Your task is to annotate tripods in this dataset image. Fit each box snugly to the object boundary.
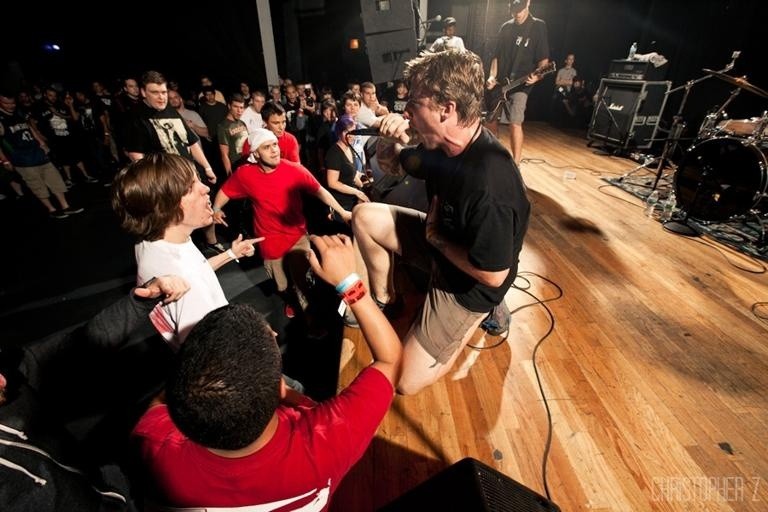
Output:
[616,64,733,192]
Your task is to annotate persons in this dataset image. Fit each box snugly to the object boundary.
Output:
[132,232,404,512]
[325,116,371,232]
[115,75,140,120]
[284,76,335,128]
[211,129,353,319]
[114,71,227,254]
[243,101,301,165]
[266,83,282,111]
[112,152,304,393]
[553,54,578,115]
[389,79,412,114]
[198,75,226,103]
[353,50,530,396]
[0,273,192,512]
[335,93,361,138]
[167,89,208,138]
[235,78,253,107]
[429,16,465,52]
[215,94,250,177]
[168,81,178,91]
[240,92,266,145]
[197,86,229,136]
[355,81,388,129]
[0,70,112,220]
[484,0,550,166]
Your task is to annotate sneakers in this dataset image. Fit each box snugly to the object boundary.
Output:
[484,300,511,332]
[66,178,75,185]
[62,205,84,215]
[284,303,295,319]
[83,175,99,183]
[49,211,69,219]
[207,242,226,255]
[342,291,407,328]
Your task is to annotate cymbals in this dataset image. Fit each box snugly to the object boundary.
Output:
[704,67,768,104]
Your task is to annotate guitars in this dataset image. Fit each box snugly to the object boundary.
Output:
[484,60,556,123]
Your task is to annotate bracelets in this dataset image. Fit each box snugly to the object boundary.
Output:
[212,208,221,211]
[204,167,213,171]
[337,273,359,293]
[338,281,368,304]
[227,248,237,260]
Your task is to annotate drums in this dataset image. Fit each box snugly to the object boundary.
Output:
[673,135,768,225]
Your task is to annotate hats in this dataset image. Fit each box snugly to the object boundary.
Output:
[506,1,530,13]
[440,16,456,26]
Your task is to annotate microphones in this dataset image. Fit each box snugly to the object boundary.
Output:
[421,14,442,24]
[348,127,420,146]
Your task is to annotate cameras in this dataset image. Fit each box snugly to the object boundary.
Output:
[304,89,315,107]
